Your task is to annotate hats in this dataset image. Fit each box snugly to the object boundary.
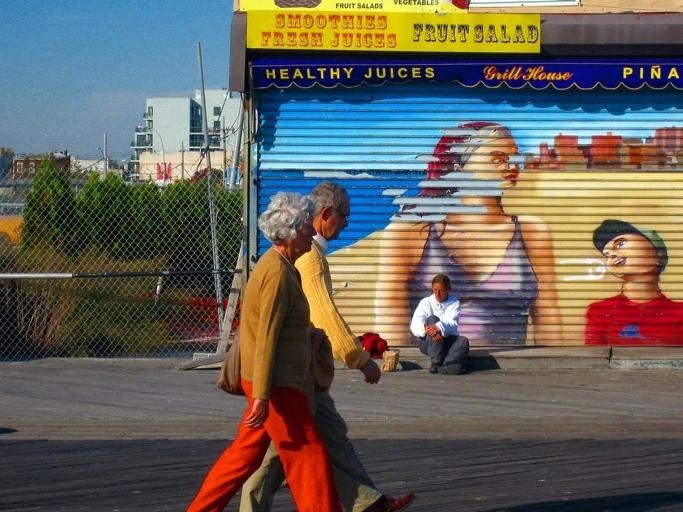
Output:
[593,219,668,274]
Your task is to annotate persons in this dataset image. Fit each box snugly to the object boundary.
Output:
[374,122,564,348]
[182,192,343,512]
[409,275,469,375]
[584,217,683,349]
[239,183,417,512]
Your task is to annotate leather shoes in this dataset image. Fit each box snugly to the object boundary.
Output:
[429,362,442,373]
[363,493,416,512]
[438,361,461,374]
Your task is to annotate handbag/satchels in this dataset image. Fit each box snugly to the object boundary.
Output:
[381,350,400,372]
[217,327,334,396]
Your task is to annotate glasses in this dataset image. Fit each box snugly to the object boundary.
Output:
[327,206,352,223]
